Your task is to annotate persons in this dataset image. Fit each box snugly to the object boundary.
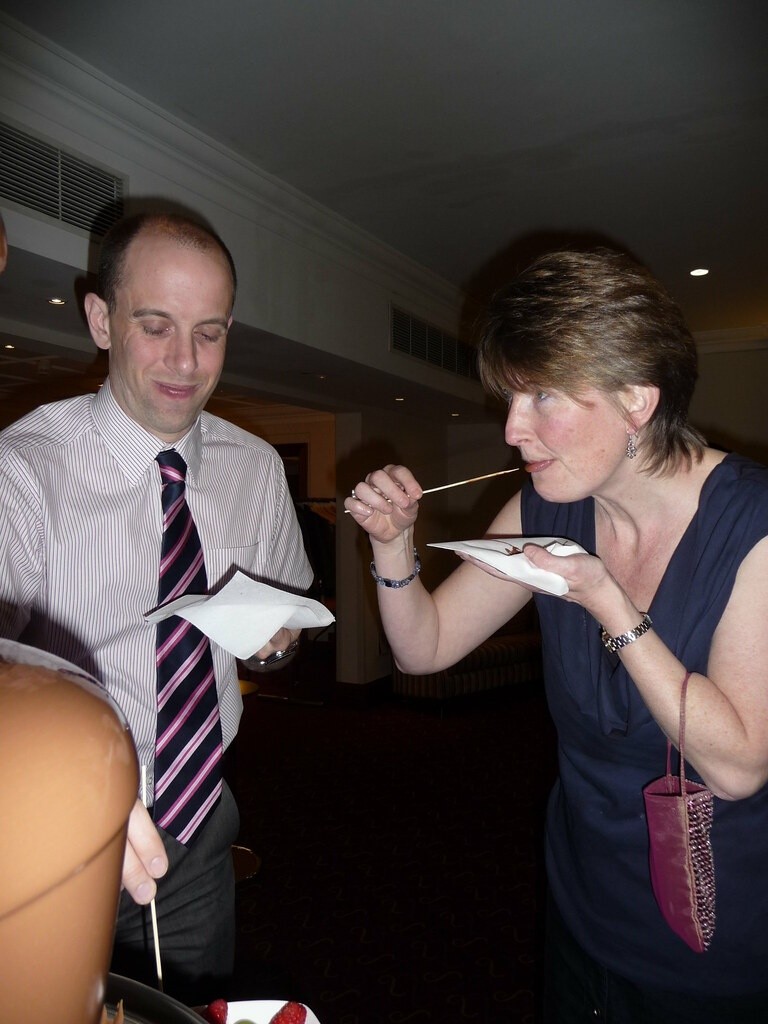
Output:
[0,214,314,1002]
[344,242,768,1024]
[0,655,138,1024]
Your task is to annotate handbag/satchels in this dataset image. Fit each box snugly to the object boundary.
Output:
[645,774,718,955]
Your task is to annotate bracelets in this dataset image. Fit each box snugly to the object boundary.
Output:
[600,613,650,652]
[369,547,420,586]
[273,649,287,657]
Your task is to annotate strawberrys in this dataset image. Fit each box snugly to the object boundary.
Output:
[268,1001,306,1024]
[200,999,228,1024]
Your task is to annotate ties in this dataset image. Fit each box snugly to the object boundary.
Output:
[152,451,225,849]
